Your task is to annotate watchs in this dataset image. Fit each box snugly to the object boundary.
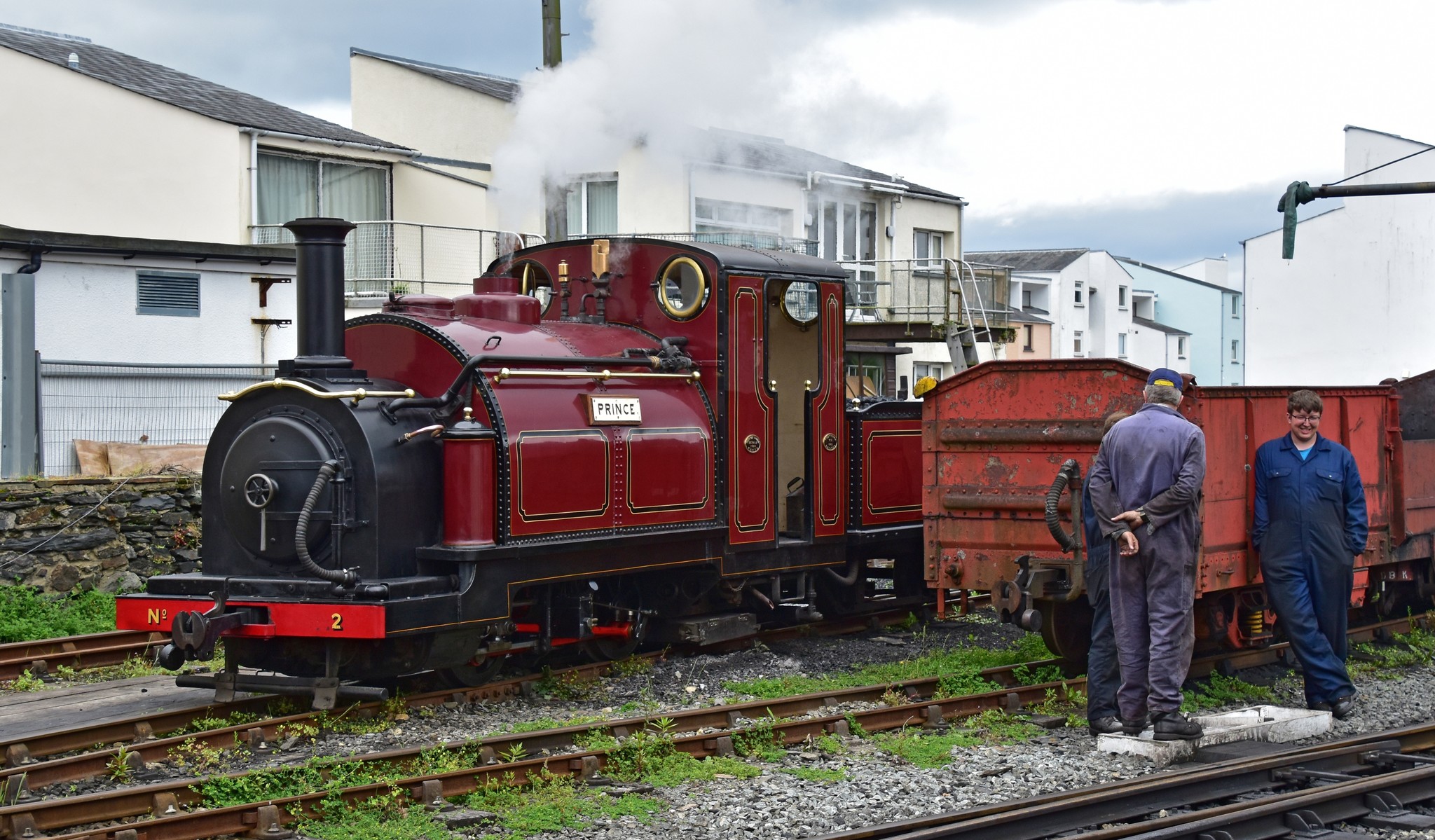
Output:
[1140,510,1150,523]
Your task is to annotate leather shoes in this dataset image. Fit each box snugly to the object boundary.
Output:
[1122,717,1148,733]
[1153,710,1204,740]
[1089,715,1123,735]
[1309,689,1359,715]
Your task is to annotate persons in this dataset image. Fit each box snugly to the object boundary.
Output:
[1081,410,1132,732]
[1255,389,1369,721]
[1090,367,1204,741]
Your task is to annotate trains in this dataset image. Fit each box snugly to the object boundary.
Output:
[115,214,934,714]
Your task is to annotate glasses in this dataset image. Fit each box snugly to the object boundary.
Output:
[1290,413,1321,422]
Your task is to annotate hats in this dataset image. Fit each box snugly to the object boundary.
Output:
[1147,367,1183,391]
